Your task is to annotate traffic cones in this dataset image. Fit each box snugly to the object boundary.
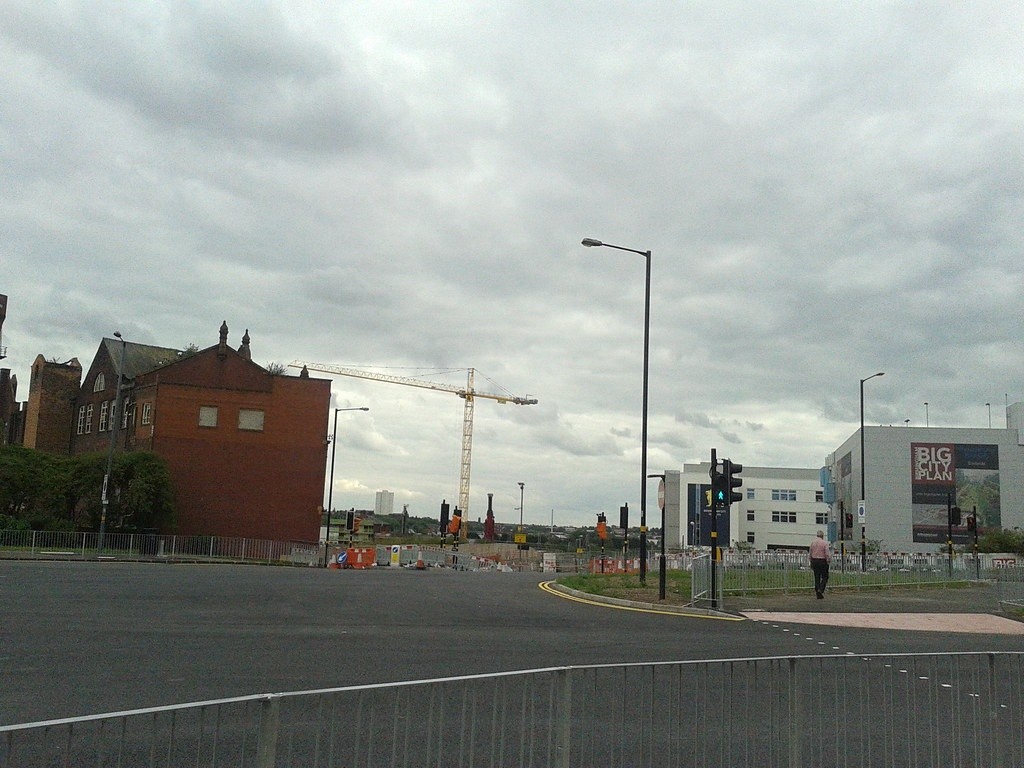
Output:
[416,552,426,570]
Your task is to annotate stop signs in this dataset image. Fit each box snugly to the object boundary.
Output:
[658,480,664,509]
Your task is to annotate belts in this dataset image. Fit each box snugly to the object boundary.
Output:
[811,559,826,561]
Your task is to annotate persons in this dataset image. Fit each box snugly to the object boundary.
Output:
[808,531,830,599]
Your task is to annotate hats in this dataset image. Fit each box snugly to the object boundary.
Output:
[817,530,825,536]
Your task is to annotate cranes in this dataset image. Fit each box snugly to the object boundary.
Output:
[287,358,538,540]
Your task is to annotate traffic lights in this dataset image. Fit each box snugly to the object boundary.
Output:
[967,517,974,530]
[715,474,728,506]
[846,514,853,528]
[728,458,742,504]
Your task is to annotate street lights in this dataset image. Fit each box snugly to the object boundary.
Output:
[860,372,885,572]
[581,237,652,584]
[97,331,127,553]
[985,402,991,428]
[324,407,369,567]
[517,482,525,564]
[924,402,928,426]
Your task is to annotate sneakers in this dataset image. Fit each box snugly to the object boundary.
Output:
[815,590,824,599]
